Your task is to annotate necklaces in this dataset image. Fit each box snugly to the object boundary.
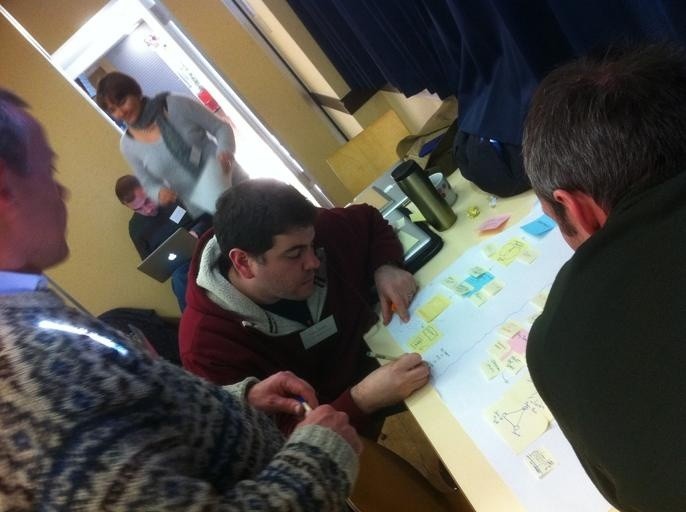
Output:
[129,119,158,133]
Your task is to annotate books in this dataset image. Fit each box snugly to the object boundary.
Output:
[354,186,394,216]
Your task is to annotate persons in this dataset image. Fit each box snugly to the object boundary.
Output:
[174,170,460,490]
[114,174,214,315]
[94,70,252,222]
[517,41,686,510]
[0,86,367,510]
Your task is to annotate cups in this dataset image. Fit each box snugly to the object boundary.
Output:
[391,160,456,231]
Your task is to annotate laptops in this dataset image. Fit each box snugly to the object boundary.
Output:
[381,209,431,264]
[137,227,198,283]
[352,159,412,219]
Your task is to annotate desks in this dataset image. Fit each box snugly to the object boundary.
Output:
[342,156,619,511]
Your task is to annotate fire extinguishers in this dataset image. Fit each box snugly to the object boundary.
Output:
[197,87,220,112]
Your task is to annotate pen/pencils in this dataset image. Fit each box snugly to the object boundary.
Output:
[299,397,312,412]
[366,352,397,360]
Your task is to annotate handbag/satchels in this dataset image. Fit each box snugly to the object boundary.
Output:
[445,0,540,200]
